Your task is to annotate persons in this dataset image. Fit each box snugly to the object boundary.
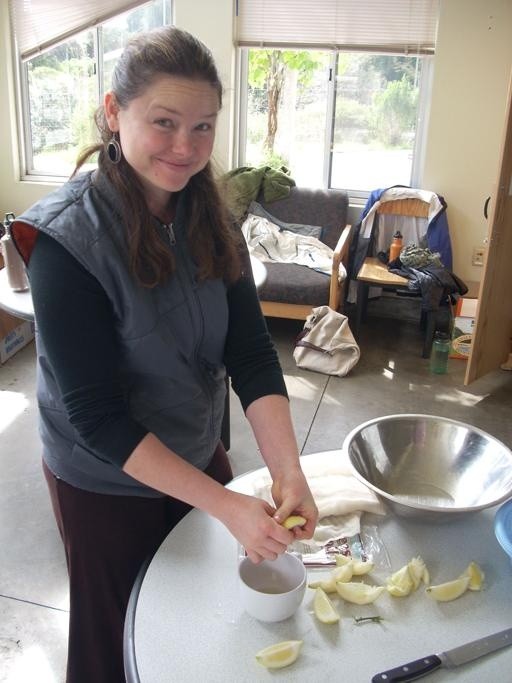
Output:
[8,29,320,683]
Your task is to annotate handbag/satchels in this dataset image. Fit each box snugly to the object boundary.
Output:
[292,305,361,377]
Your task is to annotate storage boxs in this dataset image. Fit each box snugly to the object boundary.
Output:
[447,280,482,359]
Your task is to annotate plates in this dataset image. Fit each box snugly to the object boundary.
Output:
[492,498,512,559]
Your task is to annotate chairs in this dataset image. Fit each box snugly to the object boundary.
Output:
[358,198,438,358]
[237,185,352,319]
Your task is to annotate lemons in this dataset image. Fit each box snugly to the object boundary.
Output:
[255,516,486,670]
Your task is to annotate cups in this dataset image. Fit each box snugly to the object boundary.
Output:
[235,551,308,624]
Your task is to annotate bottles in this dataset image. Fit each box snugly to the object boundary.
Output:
[1,210,30,292]
[389,230,403,262]
[429,332,450,375]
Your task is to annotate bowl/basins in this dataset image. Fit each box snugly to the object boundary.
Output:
[342,411,511,520]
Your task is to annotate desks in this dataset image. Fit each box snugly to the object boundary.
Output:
[1,266,35,320]
[121,448,511,681]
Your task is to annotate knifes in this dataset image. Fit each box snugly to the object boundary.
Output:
[372,626,511,682]
